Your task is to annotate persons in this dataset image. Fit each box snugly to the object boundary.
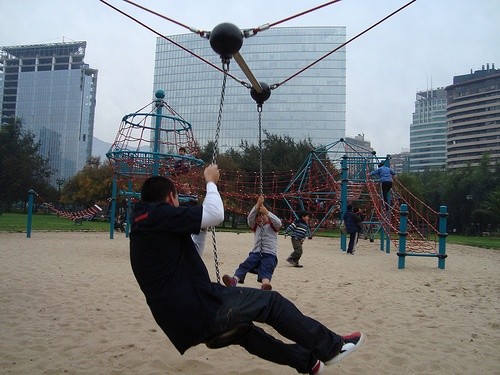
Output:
[367,163,395,211]
[171,147,198,173]
[114,213,126,233]
[344,204,362,256]
[223,196,282,290]
[284,211,313,267]
[129,164,363,375]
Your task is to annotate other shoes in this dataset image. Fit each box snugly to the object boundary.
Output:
[293,265,303,267]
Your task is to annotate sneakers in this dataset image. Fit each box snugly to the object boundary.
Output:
[287,258,295,265]
[324,333,363,365]
[310,360,324,375]
[261,284,272,291]
[222,274,237,287]
